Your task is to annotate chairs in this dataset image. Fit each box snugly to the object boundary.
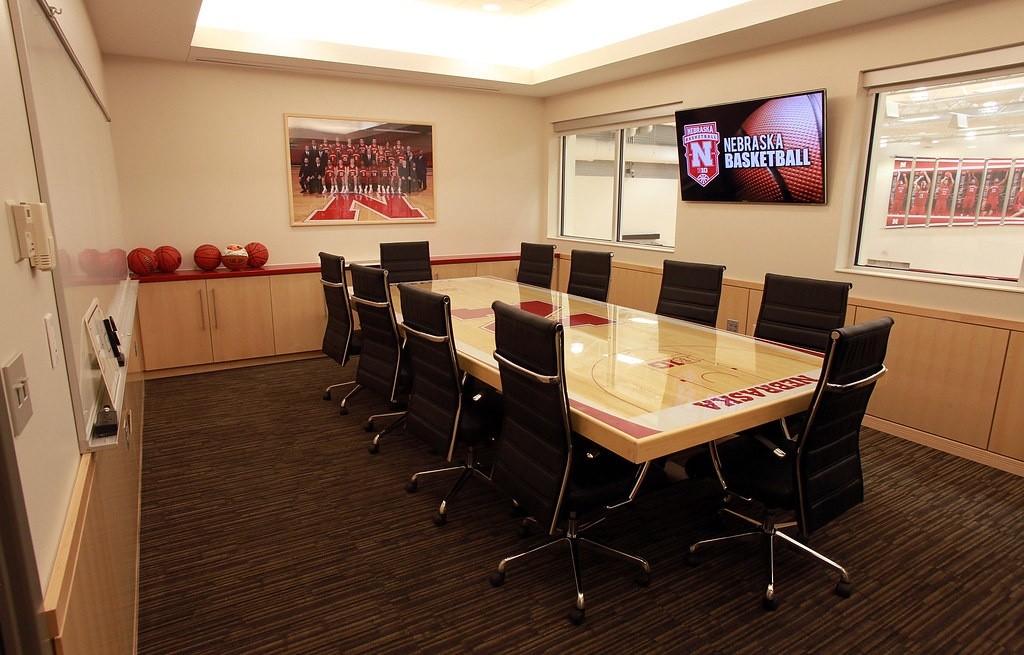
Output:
[656,260,726,327]
[688,316,894,612]
[754,273,852,352]
[567,250,615,302]
[517,243,556,288]
[349,263,410,453]
[318,252,364,415]
[380,241,432,283]
[492,300,650,625]
[397,282,525,524]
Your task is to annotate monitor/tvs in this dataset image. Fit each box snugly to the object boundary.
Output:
[675,88,828,206]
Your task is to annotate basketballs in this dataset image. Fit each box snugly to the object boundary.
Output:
[154,245,183,273]
[220,243,250,271]
[193,244,222,271]
[58,246,127,279]
[244,241,269,268]
[919,170,926,180]
[127,247,158,275]
[944,171,950,180]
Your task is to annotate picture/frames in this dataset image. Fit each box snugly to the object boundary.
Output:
[284,113,436,226]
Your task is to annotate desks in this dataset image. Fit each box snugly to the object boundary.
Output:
[348,275,826,509]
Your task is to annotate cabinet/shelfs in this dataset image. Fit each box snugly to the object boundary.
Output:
[132,252,521,382]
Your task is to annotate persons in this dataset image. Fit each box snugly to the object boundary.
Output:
[892,172,1024,219]
[298,136,428,195]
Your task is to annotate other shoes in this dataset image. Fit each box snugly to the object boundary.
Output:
[300,189,418,194]
